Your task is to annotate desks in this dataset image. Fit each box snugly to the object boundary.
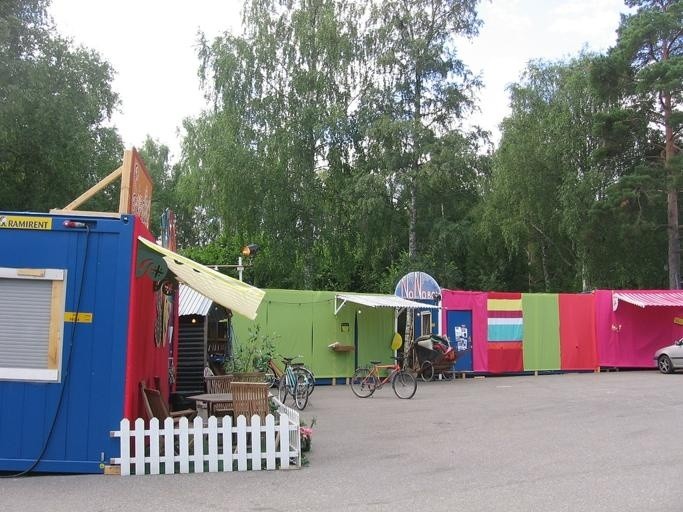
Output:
[184,392,273,453]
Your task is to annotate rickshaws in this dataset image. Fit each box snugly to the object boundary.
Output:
[412,335,474,381]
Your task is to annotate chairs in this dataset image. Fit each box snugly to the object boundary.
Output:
[139,375,198,456]
[203,371,279,445]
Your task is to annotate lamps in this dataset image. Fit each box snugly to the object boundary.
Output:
[241,244,258,265]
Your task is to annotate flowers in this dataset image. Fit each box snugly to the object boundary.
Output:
[298,424,313,440]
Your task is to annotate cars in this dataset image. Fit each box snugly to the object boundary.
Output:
[653,336,683,374]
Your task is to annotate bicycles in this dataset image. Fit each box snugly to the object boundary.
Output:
[259,349,316,411]
[351,355,417,399]
